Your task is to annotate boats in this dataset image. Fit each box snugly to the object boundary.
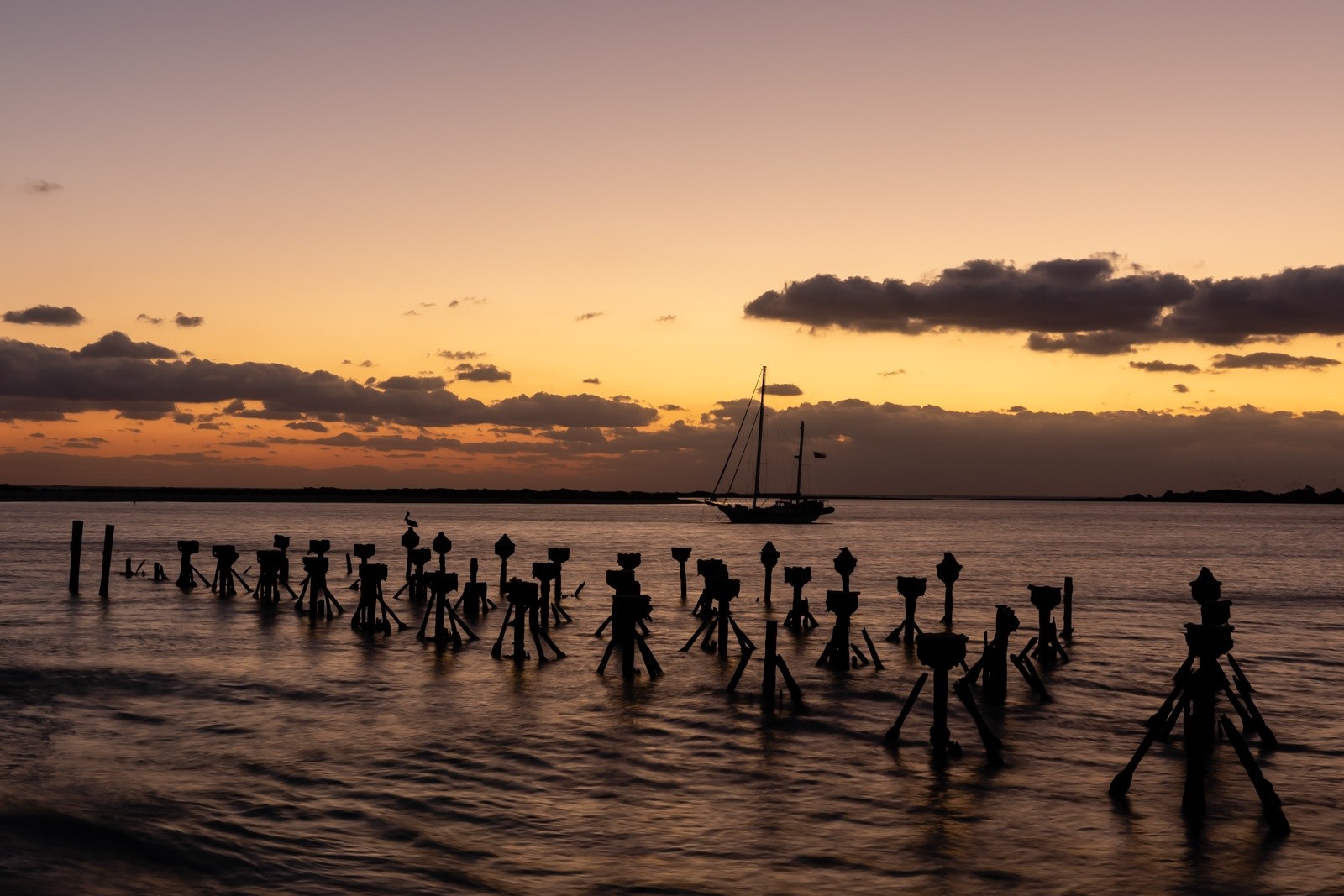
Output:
[703,367,835,524]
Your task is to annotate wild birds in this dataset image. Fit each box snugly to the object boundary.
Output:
[403,512,419,528]
